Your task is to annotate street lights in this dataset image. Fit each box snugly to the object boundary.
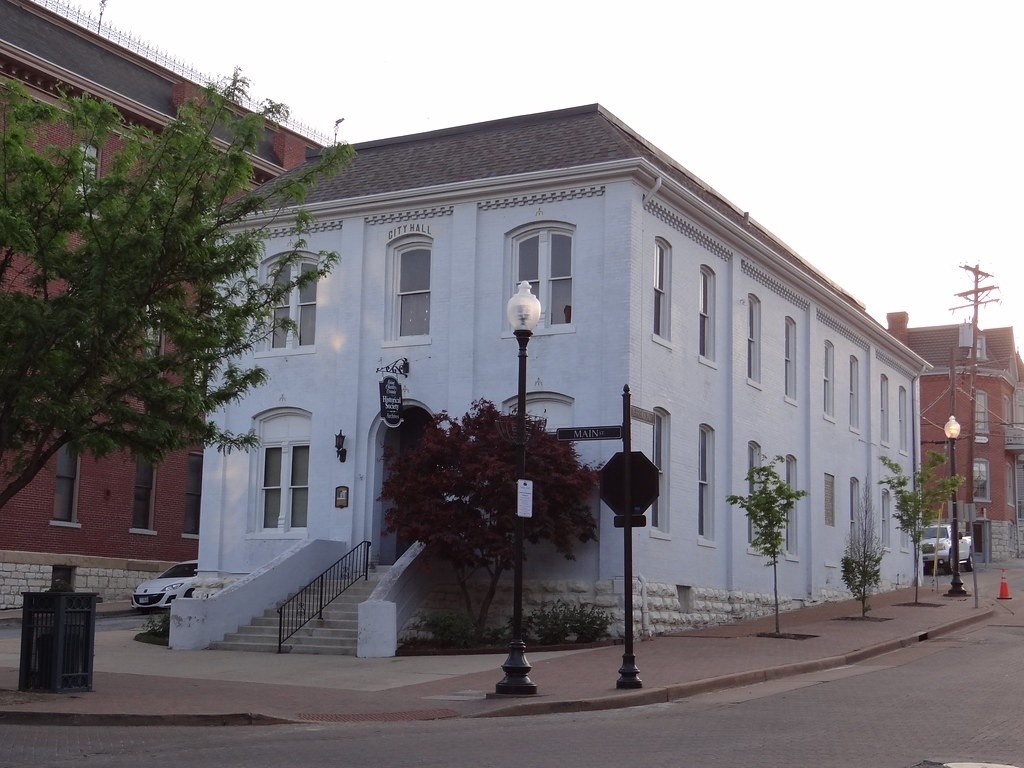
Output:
[496,281,541,695]
[943,415,972,597]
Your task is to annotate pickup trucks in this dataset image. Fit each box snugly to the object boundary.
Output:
[922,524,975,574]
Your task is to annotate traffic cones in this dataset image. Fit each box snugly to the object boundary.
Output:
[996,568,1012,599]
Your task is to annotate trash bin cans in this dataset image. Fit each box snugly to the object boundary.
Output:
[18,589,97,694]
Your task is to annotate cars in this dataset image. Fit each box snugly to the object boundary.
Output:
[130,559,199,615]
